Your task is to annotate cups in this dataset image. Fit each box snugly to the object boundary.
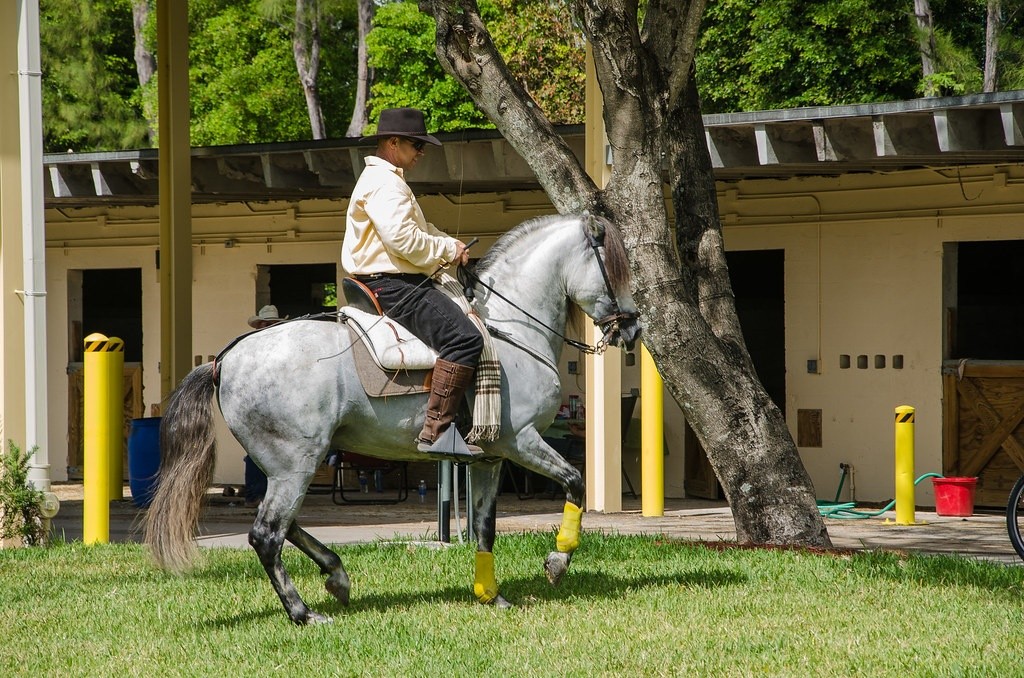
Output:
[569,394,579,419]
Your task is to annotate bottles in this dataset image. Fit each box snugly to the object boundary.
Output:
[359,471,368,493]
[576,404,585,420]
[418,480,427,504]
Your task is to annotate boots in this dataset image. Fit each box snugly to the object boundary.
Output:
[418,358,484,454]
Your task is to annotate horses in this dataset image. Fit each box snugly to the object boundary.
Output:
[126,208,645,629]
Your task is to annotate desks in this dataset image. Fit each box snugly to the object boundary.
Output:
[539,420,586,440]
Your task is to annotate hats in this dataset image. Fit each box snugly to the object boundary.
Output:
[358,108,442,147]
[248,305,287,328]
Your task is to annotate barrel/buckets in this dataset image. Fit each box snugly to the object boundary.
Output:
[127,416,163,508]
[931,478,977,516]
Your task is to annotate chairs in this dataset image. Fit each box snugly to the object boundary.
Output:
[332,448,409,506]
[556,391,637,500]
[455,459,537,502]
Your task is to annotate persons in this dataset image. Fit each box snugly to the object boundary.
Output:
[245,304,289,508]
[340,106,485,458]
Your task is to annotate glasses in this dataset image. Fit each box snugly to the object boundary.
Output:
[400,136,425,150]
